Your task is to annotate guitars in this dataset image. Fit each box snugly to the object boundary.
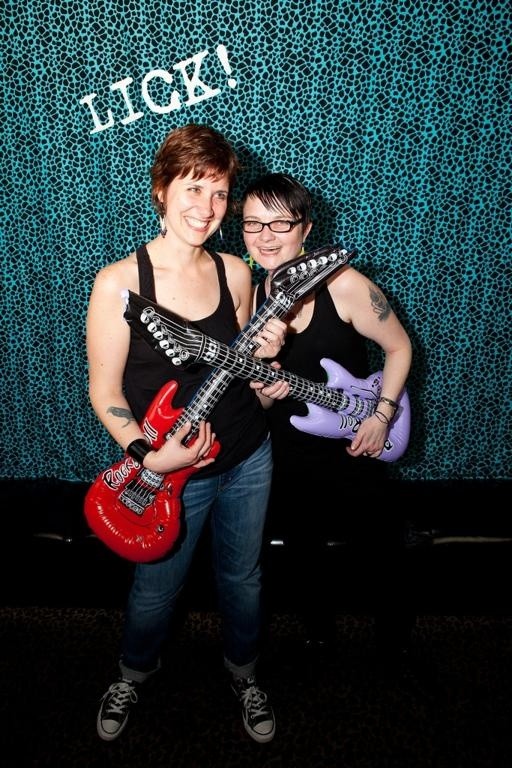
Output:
[122,288,410,462]
[85,242,359,563]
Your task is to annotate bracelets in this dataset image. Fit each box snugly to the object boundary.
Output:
[380,397,399,409]
[374,411,389,424]
[126,439,152,465]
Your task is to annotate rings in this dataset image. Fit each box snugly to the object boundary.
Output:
[199,456,202,460]
[362,452,368,456]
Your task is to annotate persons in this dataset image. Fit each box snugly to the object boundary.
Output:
[240,174,415,687]
[86,124,288,743]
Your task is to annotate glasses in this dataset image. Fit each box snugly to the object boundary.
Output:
[242,216,304,233]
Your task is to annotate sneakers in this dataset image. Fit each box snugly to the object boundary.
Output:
[97,678,138,741]
[231,675,276,742]
